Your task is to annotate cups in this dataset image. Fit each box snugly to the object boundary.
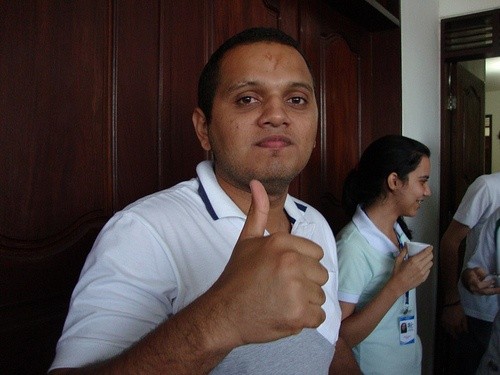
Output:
[406,242,431,257]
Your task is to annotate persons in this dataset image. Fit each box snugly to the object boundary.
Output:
[47,26,343,375]
[437,170,500,375]
[334,132,434,375]
